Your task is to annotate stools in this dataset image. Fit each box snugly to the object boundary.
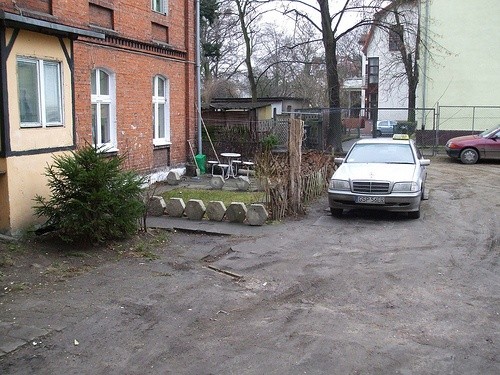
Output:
[232,160,242,176]
[243,161,254,178]
[207,160,219,176]
[217,164,229,179]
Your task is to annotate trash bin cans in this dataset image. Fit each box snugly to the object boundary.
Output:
[195,154,206,174]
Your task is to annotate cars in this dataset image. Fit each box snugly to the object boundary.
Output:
[371,120,398,137]
[444,124,500,165]
[328,133,431,219]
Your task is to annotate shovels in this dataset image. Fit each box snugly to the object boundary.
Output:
[187,140,200,176]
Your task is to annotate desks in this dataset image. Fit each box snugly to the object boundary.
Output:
[220,152,241,180]
